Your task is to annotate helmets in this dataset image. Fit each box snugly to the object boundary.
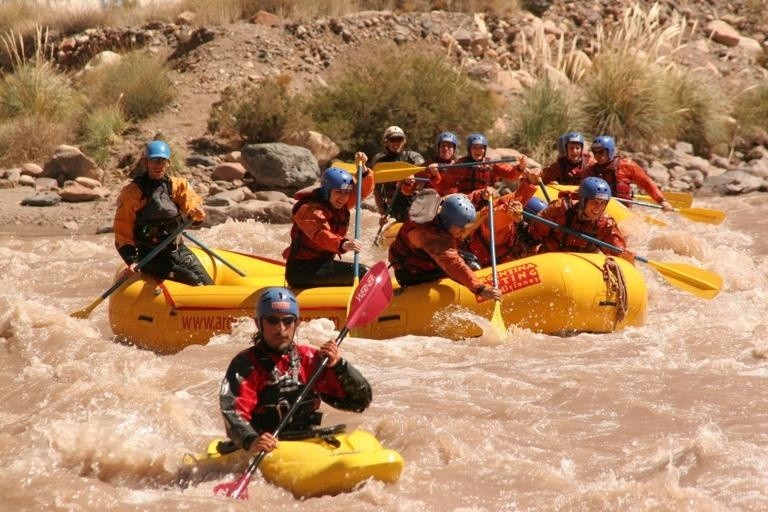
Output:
[146,141,171,160]
[321,126,616,227]
[256,287,300,324]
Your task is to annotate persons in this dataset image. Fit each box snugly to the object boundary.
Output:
[285,151,375,288]
[372,125,673,302]
[220,287,372,453]
[113,140,215,286]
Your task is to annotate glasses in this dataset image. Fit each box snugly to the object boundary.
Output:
[264,316,295,325]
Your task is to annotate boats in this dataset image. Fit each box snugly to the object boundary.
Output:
[107,247,648,355]
[378,184,706,265]
[173,424,406,500]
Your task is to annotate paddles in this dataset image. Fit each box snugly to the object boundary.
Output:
[214,262,392,498]
[346,160,366,319]
[66,217,192,320]
[488,197,506,331]
[372,160,520,184]
[517,204,723,299]
[610,190,728,232]
[332,161,433,182]
